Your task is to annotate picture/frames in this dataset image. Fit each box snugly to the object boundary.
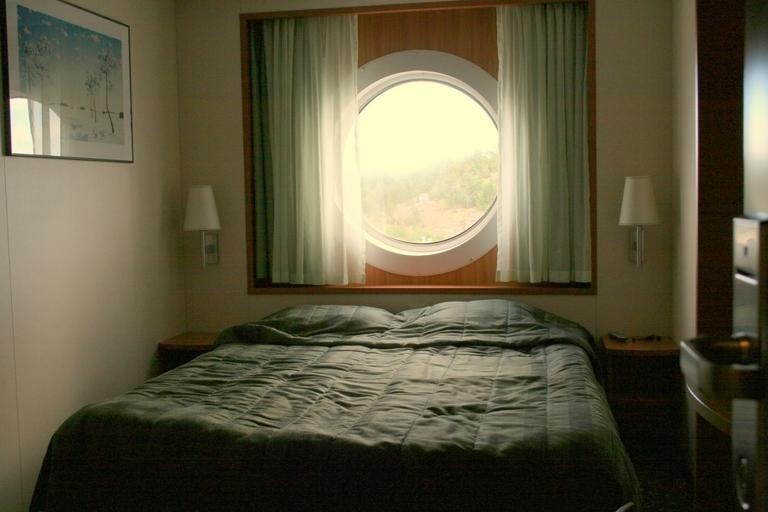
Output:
[2,1,133,163]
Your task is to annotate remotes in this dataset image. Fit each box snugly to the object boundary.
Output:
[607,331,629,342]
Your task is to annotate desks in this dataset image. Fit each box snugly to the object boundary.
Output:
[682,335,754,498]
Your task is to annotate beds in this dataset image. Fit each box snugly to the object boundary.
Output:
[78,299,596,511]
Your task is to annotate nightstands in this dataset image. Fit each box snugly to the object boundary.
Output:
[602,335,680,407]
[159,333,216,373]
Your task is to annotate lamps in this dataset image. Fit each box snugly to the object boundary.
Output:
[619,177,658,269]
[184,185,221,271]
